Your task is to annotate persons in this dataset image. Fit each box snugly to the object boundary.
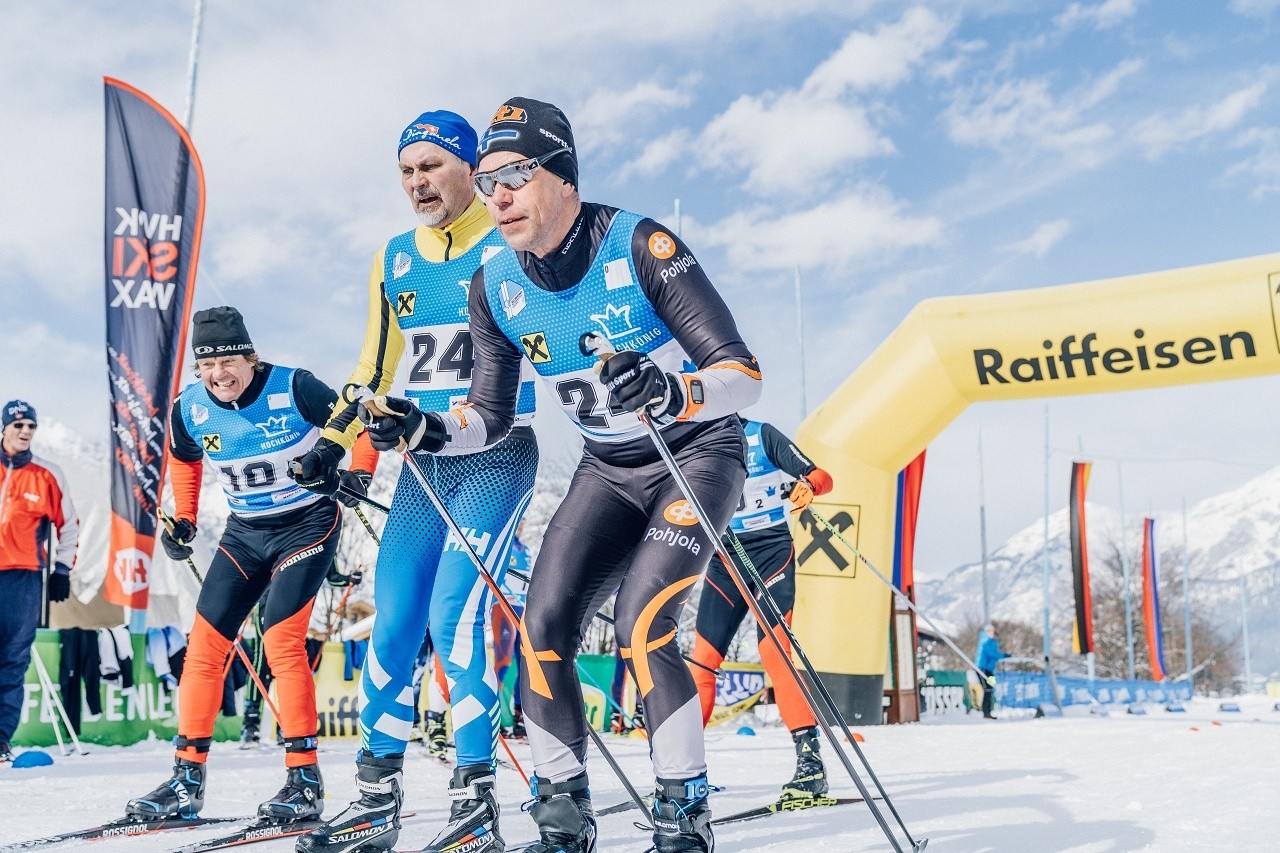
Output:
[0,401,82,763]
[238,557,646,765]
[125,305,379,825]
[287,110,538,853]
[688,417,836,800]
[358,98,762,853]
[975,623,1012,719]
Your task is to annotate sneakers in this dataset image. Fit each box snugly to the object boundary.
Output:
[523,769,597,852]
[783,725,829,797]
[652,770,714,853]
[420,763,505,853]
[0,742,17,765]
[240,700,260,749]
[257,733,325,818]
[125,734,212,816]
[294,747,404,853]
[420,710,449,762]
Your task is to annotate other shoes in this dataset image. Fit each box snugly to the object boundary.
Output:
[984,712,997,719]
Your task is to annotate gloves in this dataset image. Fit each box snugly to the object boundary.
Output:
[593,350,670,413]
[329,469,372,508]
[160,522,196,560]
[294,437,346,496]
[780,479,814,515]
[357,396,428,454]
[48,572,70,603]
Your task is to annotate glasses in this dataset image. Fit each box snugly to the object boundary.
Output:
[474,146,572,196]
[10,422,36,429]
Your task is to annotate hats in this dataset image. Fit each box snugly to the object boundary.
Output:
[984,623,999,631]
[1,400,37,432]
[192,306,255,360]
[398,110,477,168]
[476,97,579,193]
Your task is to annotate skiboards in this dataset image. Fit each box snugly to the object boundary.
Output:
[417,740,627,776]
[593,787,891,831]
[604,729,723,743]
[0,810,540,853]
[232,742,340,755]
